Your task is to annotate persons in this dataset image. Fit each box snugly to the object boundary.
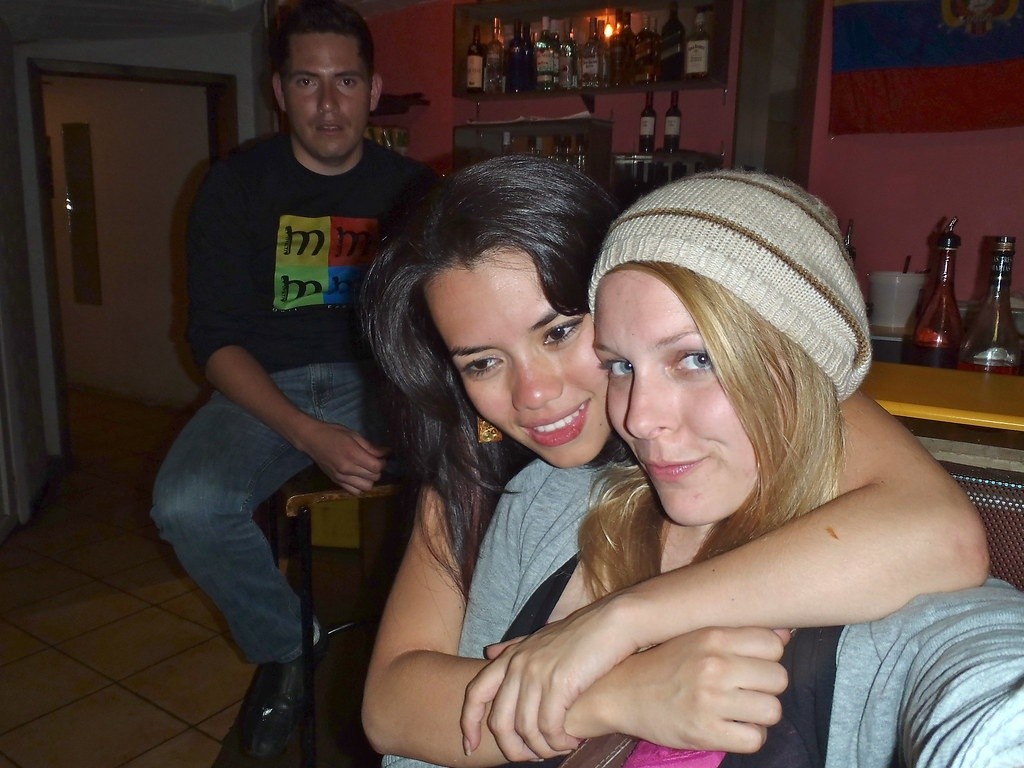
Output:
[148,0,442,768]
[355,148,990,768]
[382,167,1024,767]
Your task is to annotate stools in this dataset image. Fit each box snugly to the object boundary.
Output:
[266,464,409,768]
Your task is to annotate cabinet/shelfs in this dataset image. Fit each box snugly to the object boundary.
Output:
[453,1,732,189]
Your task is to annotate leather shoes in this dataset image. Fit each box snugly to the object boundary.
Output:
[241,626,328,757]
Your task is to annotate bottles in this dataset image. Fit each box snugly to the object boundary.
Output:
[475,131,587,173]
[956,236,1020,376]
[663,89,681,154]
[466,2,715,93]
[909,218,963,371]
[639,91,654,155]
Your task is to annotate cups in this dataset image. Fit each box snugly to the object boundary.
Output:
[868,270,926,342]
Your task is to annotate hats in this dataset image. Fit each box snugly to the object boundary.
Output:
[587,170,874,406]
